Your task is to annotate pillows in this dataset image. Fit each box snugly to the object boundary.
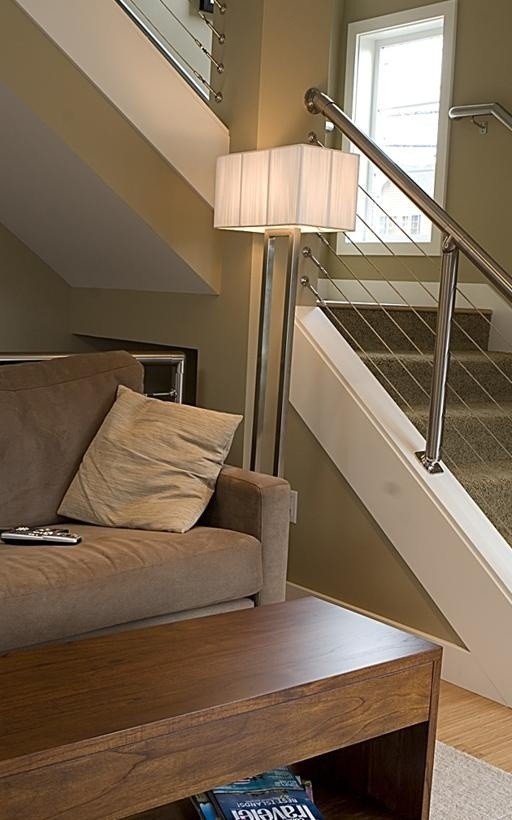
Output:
[55,382,251,538]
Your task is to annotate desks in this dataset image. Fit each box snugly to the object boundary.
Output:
[1,592,449,818]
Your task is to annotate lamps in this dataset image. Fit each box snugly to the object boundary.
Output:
[210,141,366,478]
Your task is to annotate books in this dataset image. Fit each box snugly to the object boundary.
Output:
[188,767,325,820]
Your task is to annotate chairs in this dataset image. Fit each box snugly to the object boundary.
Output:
[1,346,297,666]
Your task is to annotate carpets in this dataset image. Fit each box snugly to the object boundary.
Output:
[424,738,512,820]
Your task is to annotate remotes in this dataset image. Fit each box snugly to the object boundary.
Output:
[1,526,81,546]
